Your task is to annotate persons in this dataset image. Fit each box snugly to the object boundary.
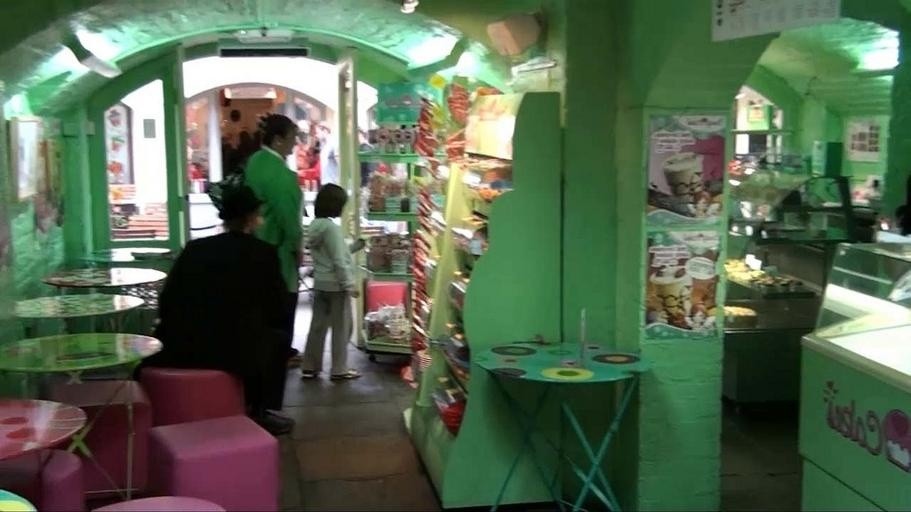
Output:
[300,184,366,380]
[244,110,303,368]
[157,187,296,434]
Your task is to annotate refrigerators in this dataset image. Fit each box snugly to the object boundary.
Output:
[799,310,910,510]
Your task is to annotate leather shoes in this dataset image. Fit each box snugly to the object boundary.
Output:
[249,410,296,436]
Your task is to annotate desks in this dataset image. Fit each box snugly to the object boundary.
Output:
[42,265,166,295]
[85,248,171,264]
[470,339,653,512]
[0,333,163,501]
[0,398,86,459]
[10,292,144,332]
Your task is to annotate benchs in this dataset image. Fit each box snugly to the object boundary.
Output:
[109,214,170,239]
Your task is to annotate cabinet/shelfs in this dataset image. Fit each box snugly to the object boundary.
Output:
[401,89,561,508]
[361,83,419,356]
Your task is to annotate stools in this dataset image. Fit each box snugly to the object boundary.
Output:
[0,446,83,511]
[151,415,281,510]
[42,383,154,491]
[85,494,223,512]
[142,365,245,421]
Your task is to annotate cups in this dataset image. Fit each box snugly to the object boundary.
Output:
[651,268,694,322]
[666,152,704,207]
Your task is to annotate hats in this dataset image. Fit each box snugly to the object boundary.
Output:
[219,185,268,220]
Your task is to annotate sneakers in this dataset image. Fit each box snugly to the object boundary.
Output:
[302,369,321,378]
[330,368,362,380]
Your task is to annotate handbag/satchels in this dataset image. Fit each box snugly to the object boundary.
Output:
[208,168,246,211]
